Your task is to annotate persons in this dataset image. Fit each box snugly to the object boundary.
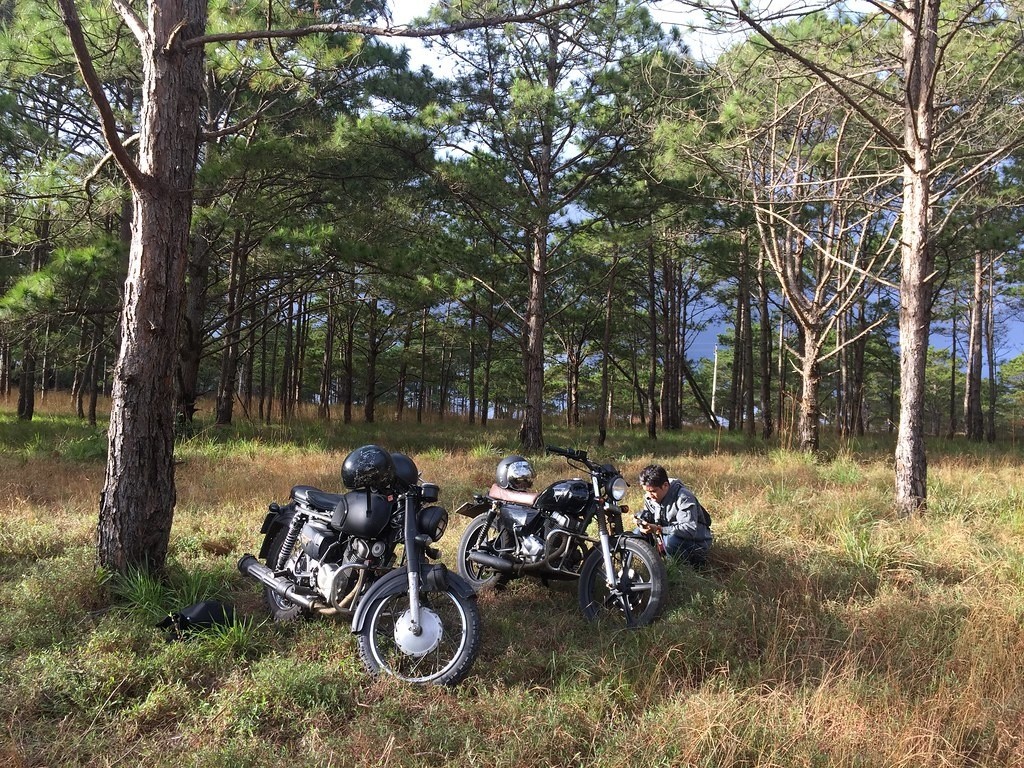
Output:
[633,465,712,568]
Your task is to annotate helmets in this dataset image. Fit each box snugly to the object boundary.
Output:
[496,455,536,492]
[389,452,420,490]
[341,443,397,493]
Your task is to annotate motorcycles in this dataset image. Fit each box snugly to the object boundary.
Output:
[232,471,483,695]
[451,446,667,634]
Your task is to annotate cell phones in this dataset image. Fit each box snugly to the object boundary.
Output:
[634,515,648,529]
